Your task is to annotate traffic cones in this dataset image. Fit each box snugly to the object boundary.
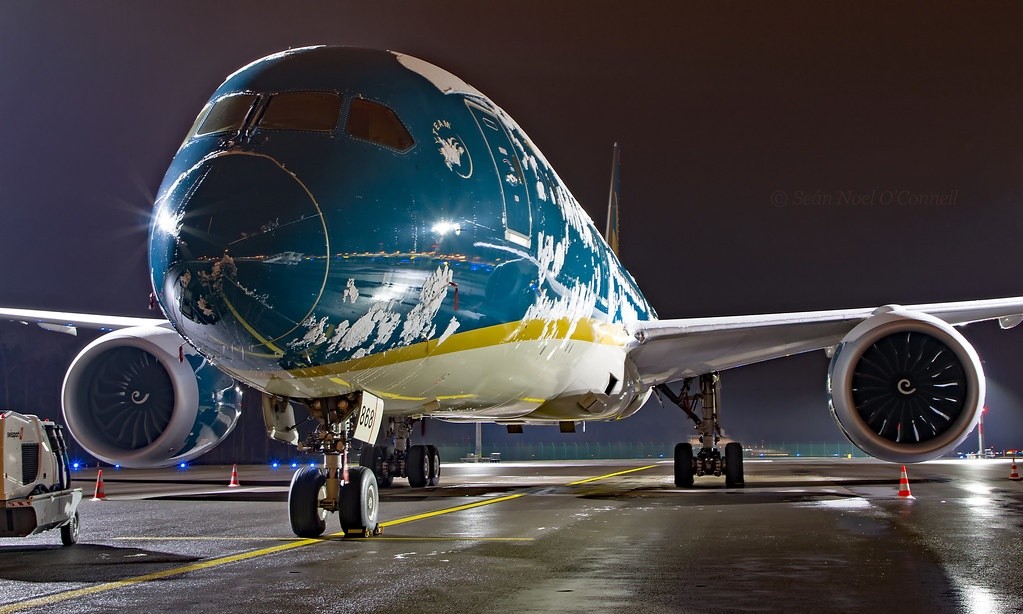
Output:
[894,465,914,499]
[91,470,108,501]
[1007,456,1023,480]
[227,462,242,487]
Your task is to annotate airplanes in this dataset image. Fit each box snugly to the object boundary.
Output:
[1,44,1023,538]
